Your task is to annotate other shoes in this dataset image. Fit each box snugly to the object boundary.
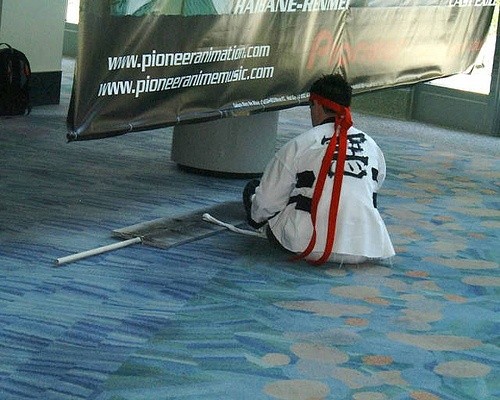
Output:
[243,179,280,228]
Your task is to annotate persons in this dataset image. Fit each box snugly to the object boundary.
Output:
[243,74,387,266]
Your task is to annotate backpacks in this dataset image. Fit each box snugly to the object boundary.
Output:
[0,43,32,115]
